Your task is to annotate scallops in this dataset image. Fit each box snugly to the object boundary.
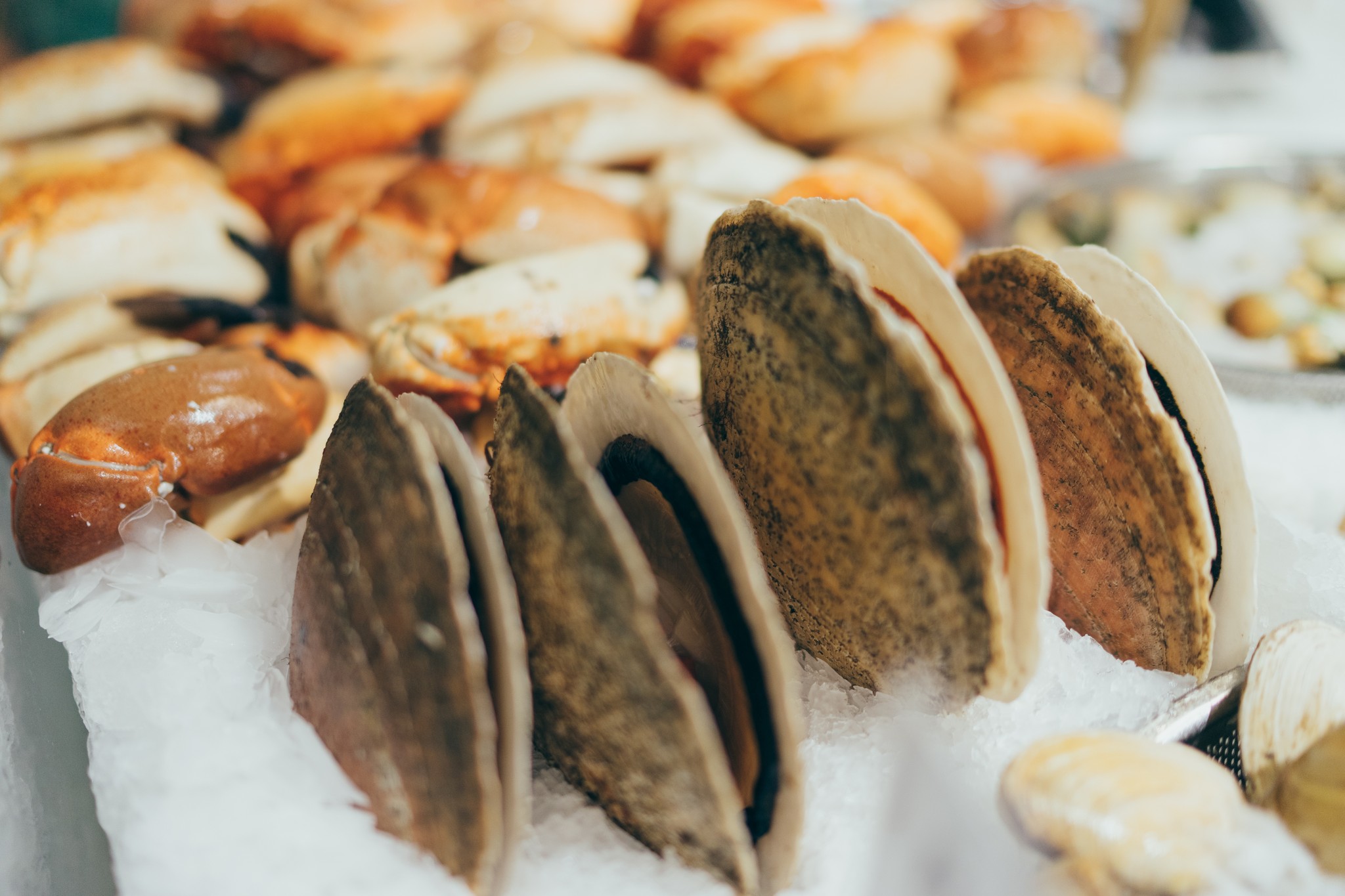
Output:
[292,198,1264,896]
